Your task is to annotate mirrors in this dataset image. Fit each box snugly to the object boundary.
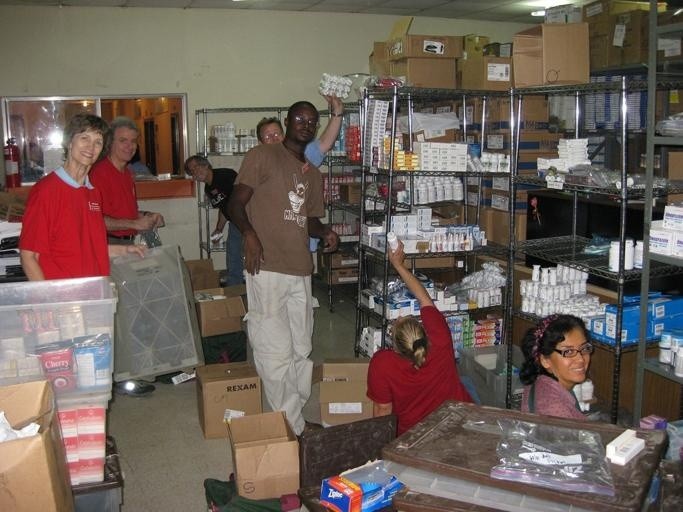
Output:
[101,96,187,178]
[7,96,98,185]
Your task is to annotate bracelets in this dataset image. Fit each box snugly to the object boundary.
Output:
[331,113,344,117]
[142,211,149,216]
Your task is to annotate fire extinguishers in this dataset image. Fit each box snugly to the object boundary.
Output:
[4,137,20,188]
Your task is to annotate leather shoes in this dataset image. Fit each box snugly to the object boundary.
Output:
[114,380,153,396]
[156,370,184,383]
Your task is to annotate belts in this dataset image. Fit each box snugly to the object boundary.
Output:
[107,234,133,241]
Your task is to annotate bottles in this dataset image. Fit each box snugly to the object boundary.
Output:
[482,152,510,173]
[212,121,258,154]
[609,241,619,272]
[467,156,476,171]
[674,346,683,379]
[625,240,634,270]
[210,233,224,241]
[634,240,644,269]
[387,232,399,253]
[318,73,352,99]
[429,232,473,253]
[473,157,484,172]
[468,287,503,309]
[397,176,462,205]
[518,262,607,322]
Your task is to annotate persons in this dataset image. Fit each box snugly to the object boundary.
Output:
[90,116,166,398]
[518,314,592,421]
[17,112,111,282]
[225,100,337,439]
[184,156,246,285]
[257,94,344,254]
[22,141,44,181]
[366,238,475,437]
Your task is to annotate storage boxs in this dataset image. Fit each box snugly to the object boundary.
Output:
[467,205,493,245]
[461,57,515,92]
[295,411,400,489]
[480,175,546,191]
[636,143,683,208]
[434,97,458,112]
[111,243,207,382]
[579,0,610,17]
[458,94,482,132]
[3,192,30,220]
[485,94,550,130]
[368,40,386,82]
[224,410,301,501]
[492,209,527,250]
[54,396,112,487]
[541,20,591,88]
[191,286,248,338]
[309,357,372,384]
[483,151,557,174]
[1,376,75,511]
[71,436,126,512]
[614,7,651,66]
[384,35,465,61]
[458,127,482,145]
[647,8,683,60]
[544,4,583,22]
[656,89,682,131]
[484,130,566,153]
[389,58,457,90]
[420,99,434,112]
[592,36,622,70]
[510,25,546,88]
[298,379,385,428]
[0,275,118,405]
[442,205,464,228]
[583,15,618,37]
[194,360,264,440]
[416,128,455,143]
[479,181,528,213]
[187,258,222,289]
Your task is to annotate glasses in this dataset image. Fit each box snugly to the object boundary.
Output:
[294,114,318,128]
[262,132,281,141]
[553,344,593,359]
[188,164,201,175]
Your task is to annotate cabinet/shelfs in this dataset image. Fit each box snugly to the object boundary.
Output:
[319,105,360,286]
[510,76,668,405]
[197,109,278,255]
[360,89,512,358]
[645,20,683,383]
